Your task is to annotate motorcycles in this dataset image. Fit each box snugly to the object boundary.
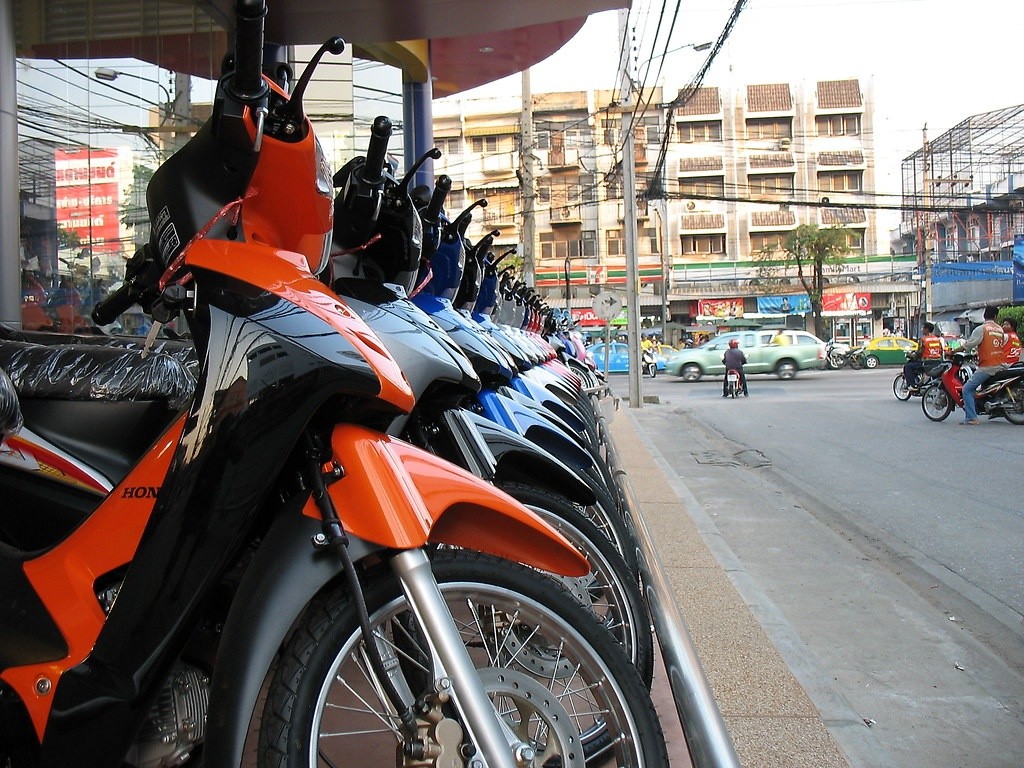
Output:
[726,368,744,399]
[824,341,866,371]
[893,349,1024,427]
[641,346,659,378]
[0,1,671,768]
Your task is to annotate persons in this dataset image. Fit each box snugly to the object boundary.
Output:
[905,323,942,391]
[940,333,944,347]
[722,339,749,397]
[640,334,656,352]
[1002,317,1022,368]
[773,328,789,346]
[780,297,793,310]
[947,305,1007,425]
[679,339,685,349]
[884,326,903,343]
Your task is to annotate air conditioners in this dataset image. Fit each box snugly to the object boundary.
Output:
[686,201,700,212]
[560,208,577,220]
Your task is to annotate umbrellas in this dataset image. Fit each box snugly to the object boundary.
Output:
[718,318,761,331]
[658,322,686,345]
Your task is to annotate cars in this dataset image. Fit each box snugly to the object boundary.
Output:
[585,342,629,373]
[651,344,680,371]
[858,332,920,369]
[664,328,827,382]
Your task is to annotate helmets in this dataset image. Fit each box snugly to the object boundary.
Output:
[641,333,649,338]
[728,339,740,348]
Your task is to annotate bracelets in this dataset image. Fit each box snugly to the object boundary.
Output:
[952,349,953,353]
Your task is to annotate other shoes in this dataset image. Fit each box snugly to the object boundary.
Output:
[745,393,749,397]
[902,385,919,392]
[959,420,979,425]
[722,394,728,397]
